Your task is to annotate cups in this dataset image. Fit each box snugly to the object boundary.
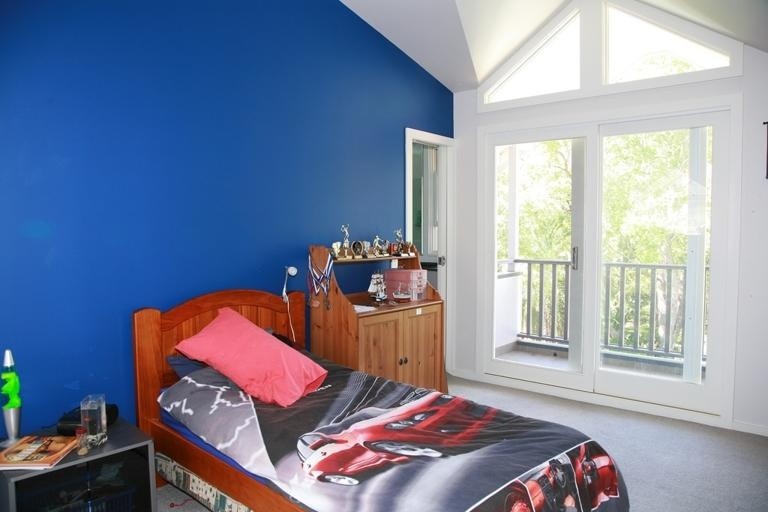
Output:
[81,395,107,447]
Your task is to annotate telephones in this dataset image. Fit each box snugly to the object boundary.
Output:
[56,398,119,436]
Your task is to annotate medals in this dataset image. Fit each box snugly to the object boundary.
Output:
[307,295,331,311]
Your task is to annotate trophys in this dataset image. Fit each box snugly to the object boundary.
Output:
[330,224,414,260]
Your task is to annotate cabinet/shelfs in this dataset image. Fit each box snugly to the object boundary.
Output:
[310,245,445,396]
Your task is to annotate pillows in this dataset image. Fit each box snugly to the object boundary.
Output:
[175,308,328,408]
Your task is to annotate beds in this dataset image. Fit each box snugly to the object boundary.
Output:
[132,290,630,512]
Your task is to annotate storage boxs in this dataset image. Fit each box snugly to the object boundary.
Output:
[384,270,427,302]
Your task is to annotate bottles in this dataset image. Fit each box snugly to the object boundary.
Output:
[76,428,89,456]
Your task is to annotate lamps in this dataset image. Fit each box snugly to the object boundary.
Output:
[282,265,298,303]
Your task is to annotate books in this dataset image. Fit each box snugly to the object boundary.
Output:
[0,434,80,471]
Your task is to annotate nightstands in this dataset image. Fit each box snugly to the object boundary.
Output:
[0,421,158,512]
